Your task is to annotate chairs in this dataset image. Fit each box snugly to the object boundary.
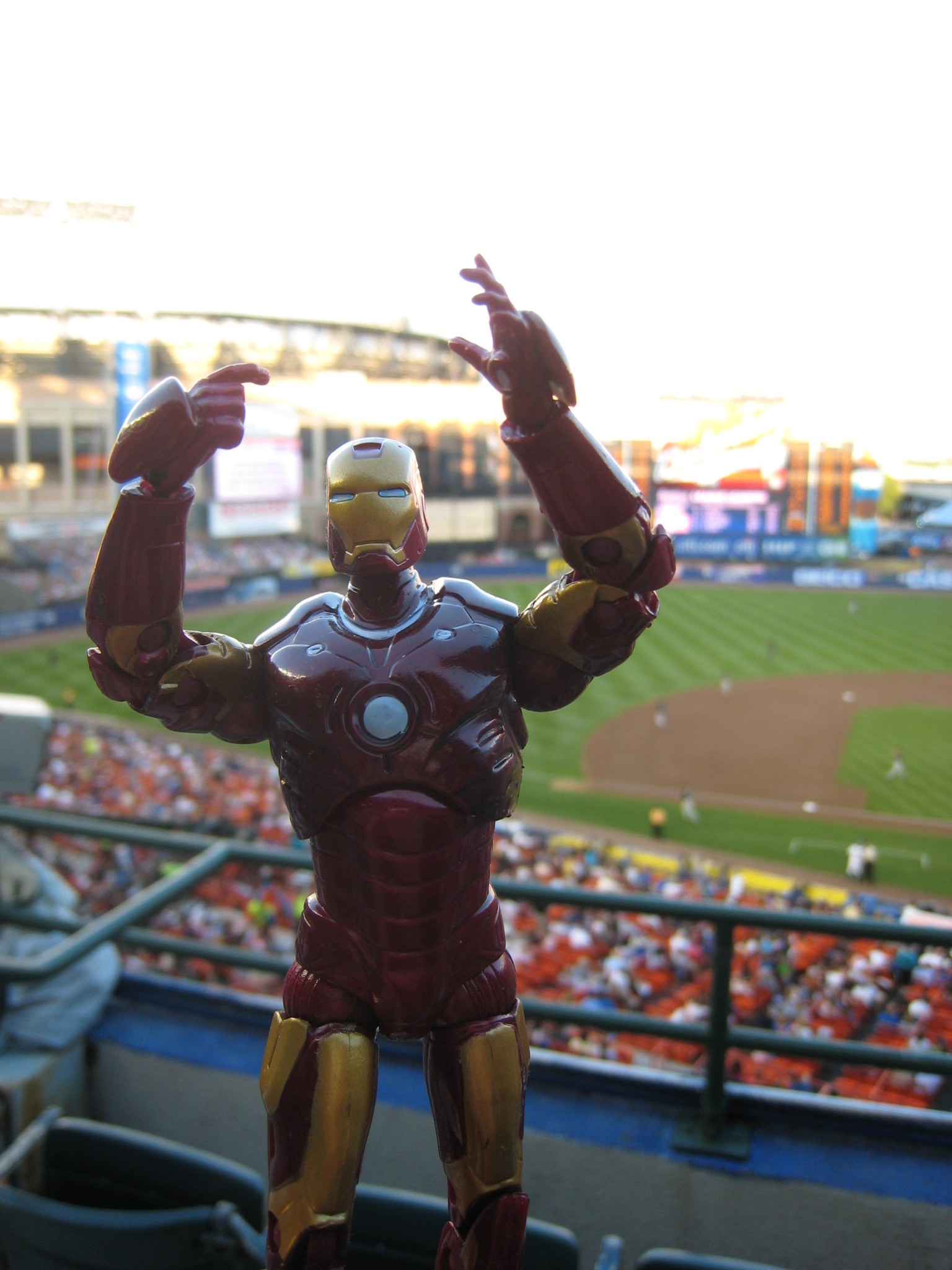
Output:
[0,1115,787,1270]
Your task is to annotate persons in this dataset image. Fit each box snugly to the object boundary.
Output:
[9,675,952,1109]
[84,256,676,1270]
